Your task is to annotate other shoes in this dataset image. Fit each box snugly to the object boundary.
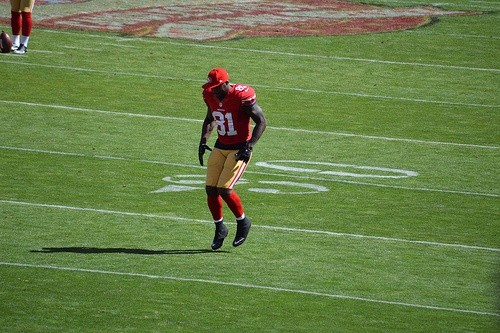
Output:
[11,42,20,50]
[13,43,28,53]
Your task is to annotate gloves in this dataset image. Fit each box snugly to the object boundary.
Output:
[235,145,253,164]
[198,143,212,166]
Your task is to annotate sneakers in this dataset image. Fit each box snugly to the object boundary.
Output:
[233,216,251,246]
[211,224,228,250]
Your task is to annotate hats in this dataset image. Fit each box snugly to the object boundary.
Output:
[202,67,229,88]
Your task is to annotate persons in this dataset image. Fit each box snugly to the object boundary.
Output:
[9,0,35,55]
[198,67,267,252]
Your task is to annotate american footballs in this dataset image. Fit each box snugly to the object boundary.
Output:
[0,31,12,52]
